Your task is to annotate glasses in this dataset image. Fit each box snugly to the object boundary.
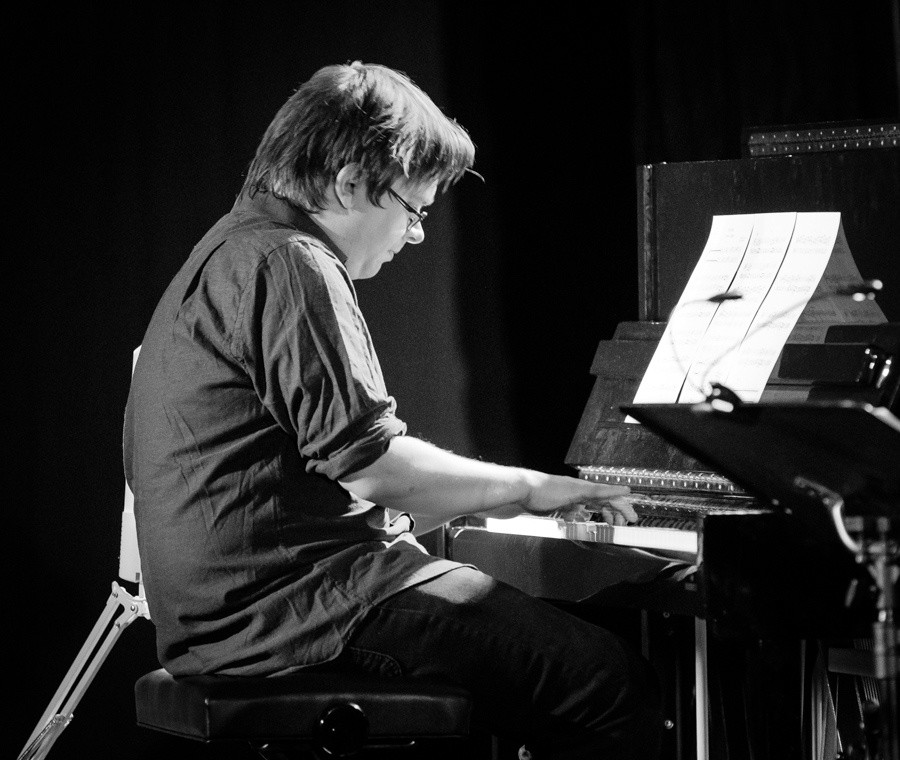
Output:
[387,188,428,229]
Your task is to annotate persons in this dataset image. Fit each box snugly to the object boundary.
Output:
[122,61,669,758]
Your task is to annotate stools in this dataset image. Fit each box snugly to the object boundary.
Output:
[134,669,477,760]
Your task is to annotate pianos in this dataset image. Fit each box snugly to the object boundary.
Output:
[436,119,900,760]
